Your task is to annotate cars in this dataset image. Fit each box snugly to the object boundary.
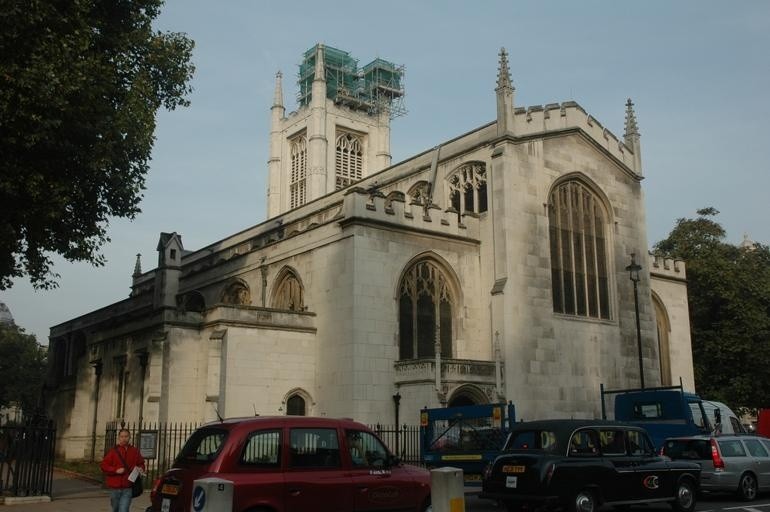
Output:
[477,418,704,512]
[145,415,431,512]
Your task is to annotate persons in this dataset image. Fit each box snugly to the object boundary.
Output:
[99,429,147,512]
[347,433,368,465]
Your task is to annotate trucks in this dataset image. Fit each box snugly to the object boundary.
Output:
[420,377,721,475]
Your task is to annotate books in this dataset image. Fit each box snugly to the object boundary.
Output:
[127,465,141,482]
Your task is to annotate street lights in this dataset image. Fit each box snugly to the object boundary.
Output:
[625,253,644,393]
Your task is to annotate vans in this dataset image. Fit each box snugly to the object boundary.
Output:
[658,435,770,501]
[634,400,748,435]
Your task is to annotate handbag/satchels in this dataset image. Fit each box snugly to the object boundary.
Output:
[132,476,142,497]
